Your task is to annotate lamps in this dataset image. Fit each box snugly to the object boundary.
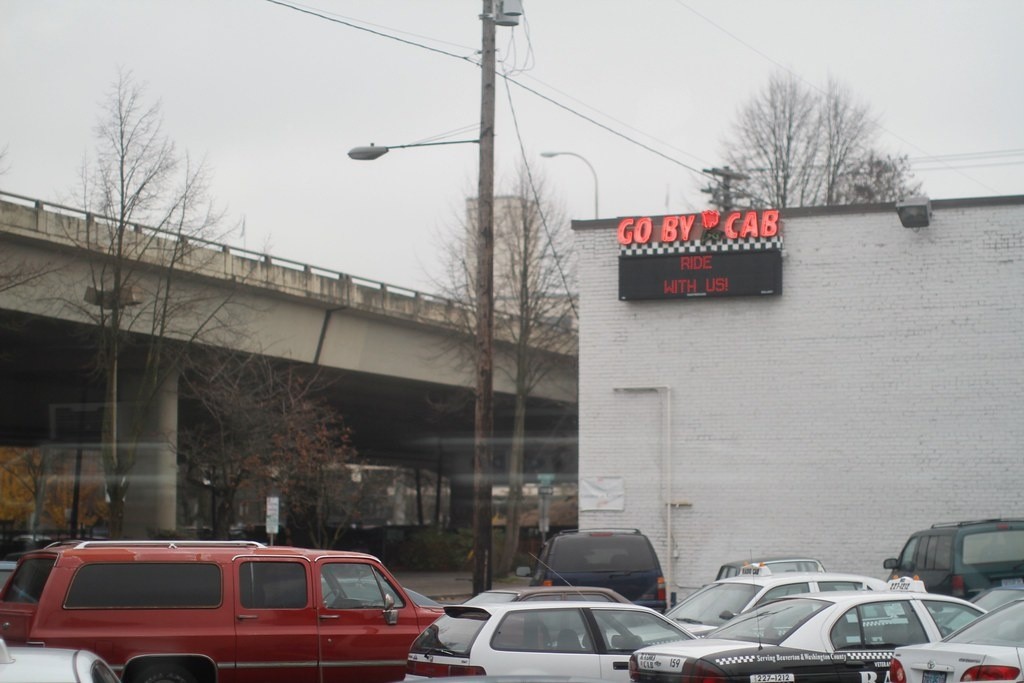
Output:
[895,197,932,228]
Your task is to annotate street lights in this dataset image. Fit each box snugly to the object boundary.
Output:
[540,150,599,219]
[346,139,494,597]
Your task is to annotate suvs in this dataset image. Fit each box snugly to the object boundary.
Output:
[403,602,699,683]
[0,541,448,683]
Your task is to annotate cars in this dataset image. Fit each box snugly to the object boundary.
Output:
[0,638,121,683]
[663,572,888,640]
[890,598,1023,683]
[628,578,989,683]
[459,587,633,606]
[938,583,1023,640]
[716,555,826,580]
[516,528,668,612]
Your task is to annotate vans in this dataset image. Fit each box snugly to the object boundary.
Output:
[882,518,1023,602]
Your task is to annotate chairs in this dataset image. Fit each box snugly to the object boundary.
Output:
[557,626,612,651]
